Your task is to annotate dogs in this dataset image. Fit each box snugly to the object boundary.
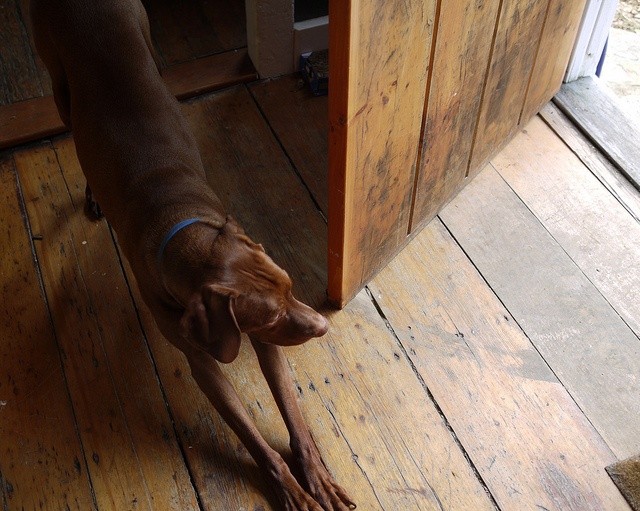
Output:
[30,0,358,511]
